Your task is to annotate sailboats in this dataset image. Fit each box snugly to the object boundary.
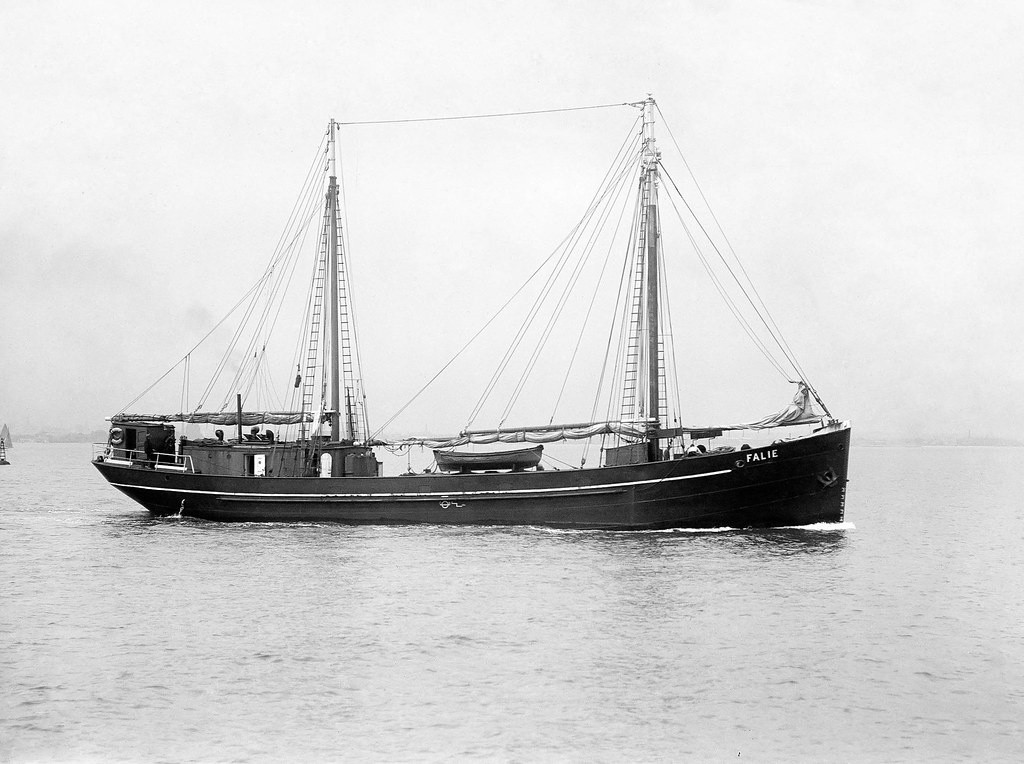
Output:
[89,92,853,538]
